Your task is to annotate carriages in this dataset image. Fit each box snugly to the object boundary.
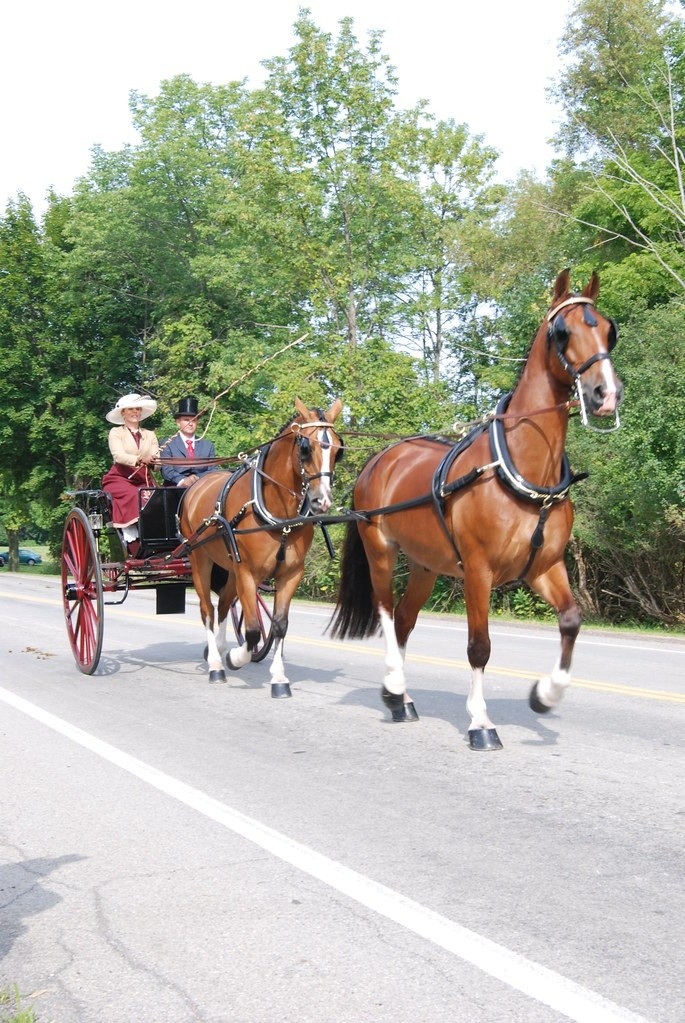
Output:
[60,266,628,751]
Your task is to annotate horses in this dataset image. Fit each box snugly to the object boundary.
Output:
[175,395,343,701]
[322,271,623,760]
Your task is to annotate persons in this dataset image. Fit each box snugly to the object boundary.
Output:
[162,398,217,487]
[101,394,160,557]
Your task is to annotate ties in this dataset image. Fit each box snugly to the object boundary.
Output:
[186,440,195,460]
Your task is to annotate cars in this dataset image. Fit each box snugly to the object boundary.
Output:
[0,557,4,567]
[0,549,42,566]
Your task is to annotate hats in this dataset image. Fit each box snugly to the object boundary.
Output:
[173,398,202,419]
[106,394,158,425]
